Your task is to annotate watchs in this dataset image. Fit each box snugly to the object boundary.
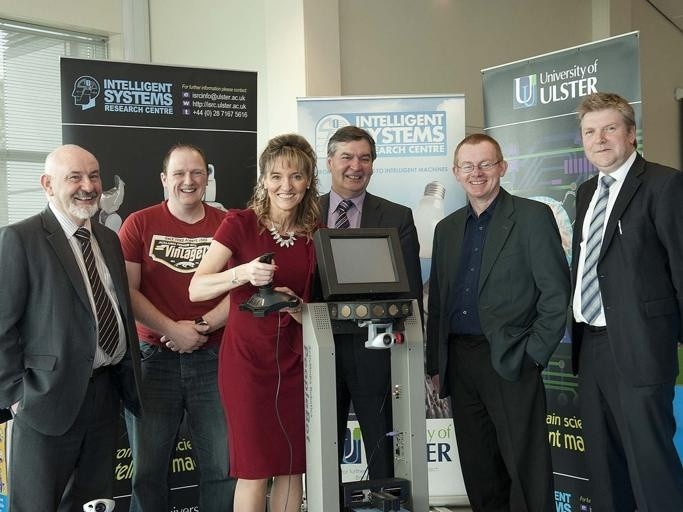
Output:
[194,316,209,336]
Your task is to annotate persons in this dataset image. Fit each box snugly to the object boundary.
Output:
[186,130,329,511]
[307,125,426,484]
[1,142,145,512]
[570,90,682,511]
[423,132,573,512]
[112,140,241,512]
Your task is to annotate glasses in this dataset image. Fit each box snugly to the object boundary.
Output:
[456,158,501,174]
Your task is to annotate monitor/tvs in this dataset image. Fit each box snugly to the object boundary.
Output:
[312,225,415,299]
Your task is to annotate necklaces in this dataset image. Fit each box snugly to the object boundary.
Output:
[265,210,299,249]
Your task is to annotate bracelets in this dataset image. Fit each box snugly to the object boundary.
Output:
[231,265,243,286]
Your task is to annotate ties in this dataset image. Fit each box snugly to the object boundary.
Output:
[73,227,120,357]
[335,198,354,230]
[581,175,615,325]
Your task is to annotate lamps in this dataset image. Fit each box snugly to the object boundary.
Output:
[358,318,394,350]
